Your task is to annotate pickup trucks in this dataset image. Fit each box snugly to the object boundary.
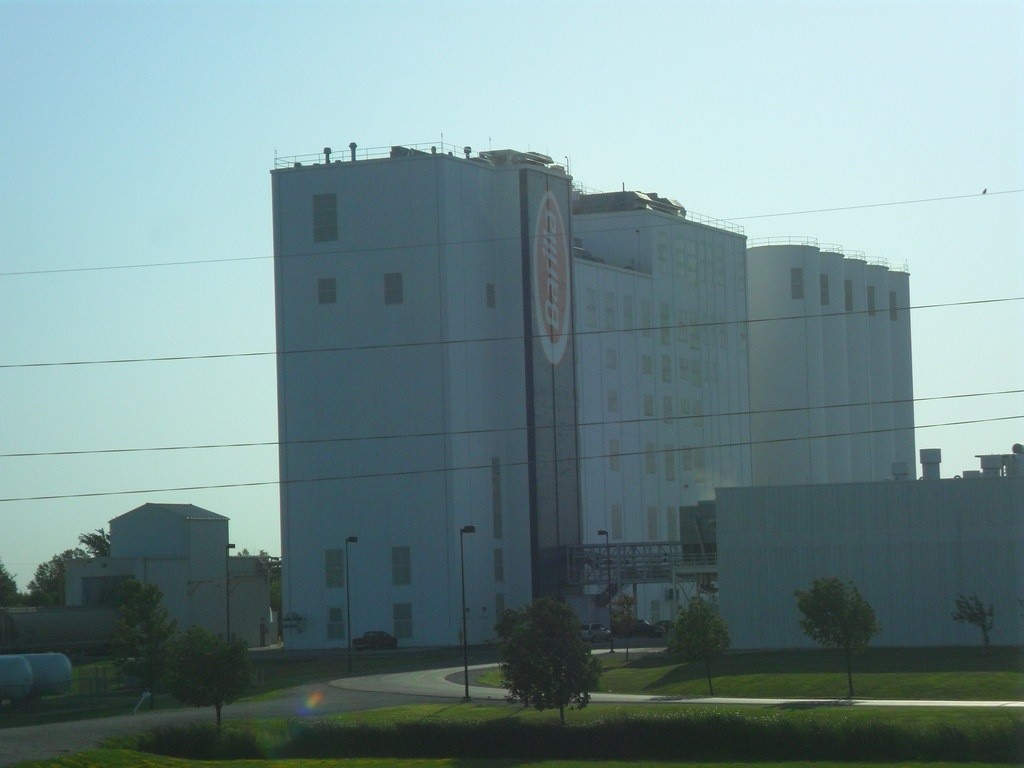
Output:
[353,630,398,650]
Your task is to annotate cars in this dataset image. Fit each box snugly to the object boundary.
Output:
[616,619,666,638]
[655,620,675,632]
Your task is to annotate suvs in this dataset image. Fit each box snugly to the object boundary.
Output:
[582,623,613,642]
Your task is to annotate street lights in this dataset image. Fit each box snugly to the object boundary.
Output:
[461,525,476,700]
[346,536,358,672]
[225,543,235,646]
[598,529,615,653]
